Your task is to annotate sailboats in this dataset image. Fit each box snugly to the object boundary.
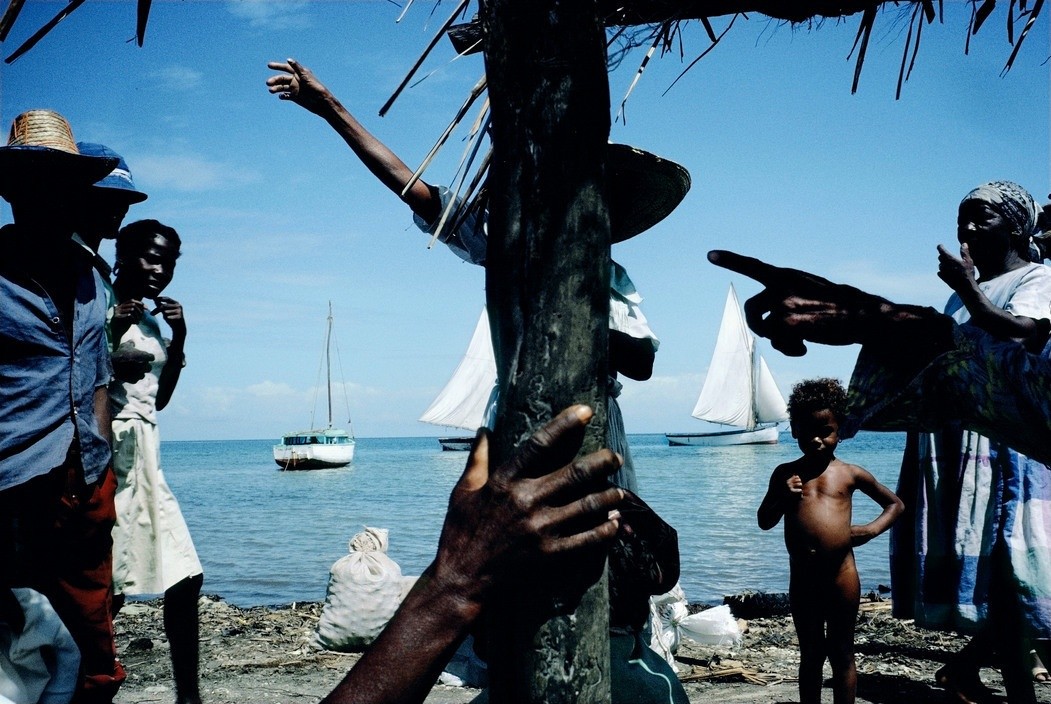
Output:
[415,306,499,452]
[271,299,355,469]
[664,280,790,447]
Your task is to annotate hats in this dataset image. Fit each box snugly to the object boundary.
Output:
[0,110,120,203]
[609,142,692,245]
[76,142,148,204]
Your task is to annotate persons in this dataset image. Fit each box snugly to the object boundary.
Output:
[708,248,1051,469]
[317,402,629,703]
[886,180,1051,704]
[264,55,692,501]
[0,109,156,704]
[107,218,204,704]
[757,377,904,704]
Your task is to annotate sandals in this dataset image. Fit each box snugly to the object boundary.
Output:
[1027,650,1048,679]
